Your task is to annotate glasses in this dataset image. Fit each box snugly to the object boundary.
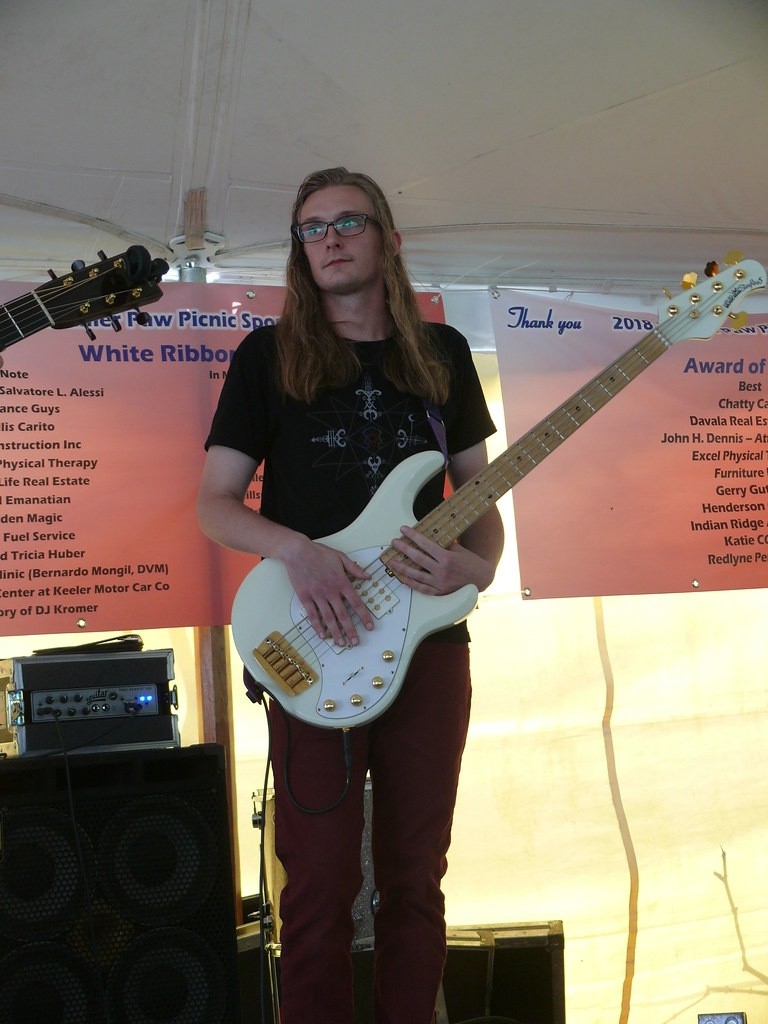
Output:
[298,214,377,243]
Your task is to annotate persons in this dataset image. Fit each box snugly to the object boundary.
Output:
[197,166,504,1024]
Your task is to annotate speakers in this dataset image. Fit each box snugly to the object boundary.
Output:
[0,743,243,1024]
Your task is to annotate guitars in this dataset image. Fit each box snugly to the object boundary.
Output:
[0,244,172,354]
[229,250,768,732]
